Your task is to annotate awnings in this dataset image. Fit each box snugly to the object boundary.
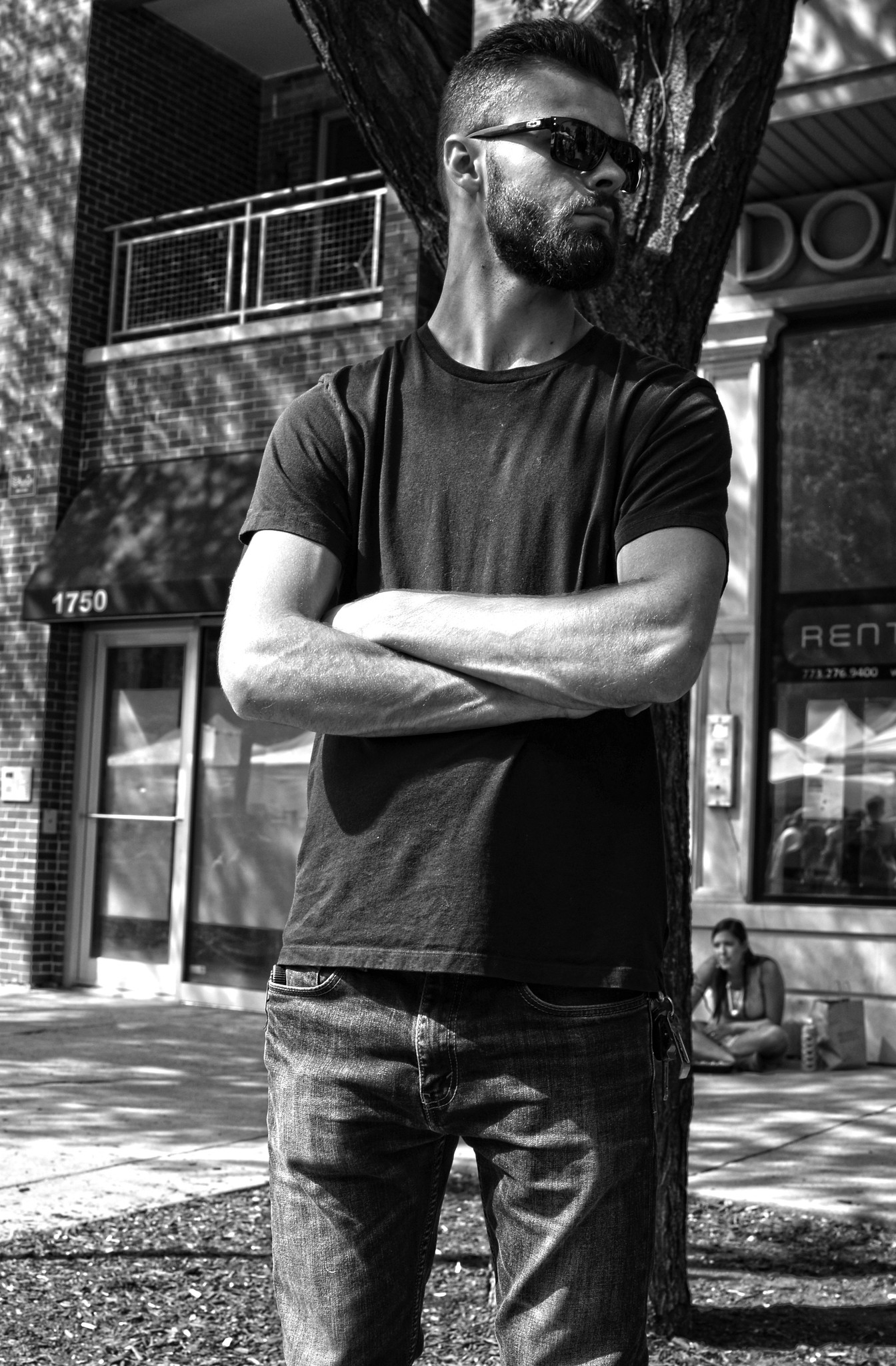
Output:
[21,461,264,622]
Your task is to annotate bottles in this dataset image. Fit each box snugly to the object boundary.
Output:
[800,1017,817,1072]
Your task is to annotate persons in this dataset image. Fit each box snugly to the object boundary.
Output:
[769,796,896,894]
[220,20,733,1366]
[690,918,787,1070]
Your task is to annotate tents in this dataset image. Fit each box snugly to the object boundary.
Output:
[107,712,316,768]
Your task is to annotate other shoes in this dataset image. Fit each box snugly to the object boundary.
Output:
[743,1050,762,1071]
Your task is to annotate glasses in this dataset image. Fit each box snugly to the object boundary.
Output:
[463,115,645,195]
